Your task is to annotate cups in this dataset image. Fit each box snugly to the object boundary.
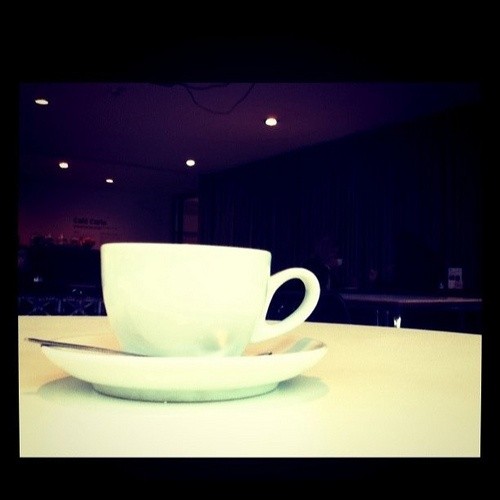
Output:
[100,242,320,356]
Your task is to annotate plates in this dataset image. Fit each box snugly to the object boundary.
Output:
[41,339,326,402]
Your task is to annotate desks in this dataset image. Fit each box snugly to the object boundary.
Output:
[336,292,483,326]
[18,315,482,458]
